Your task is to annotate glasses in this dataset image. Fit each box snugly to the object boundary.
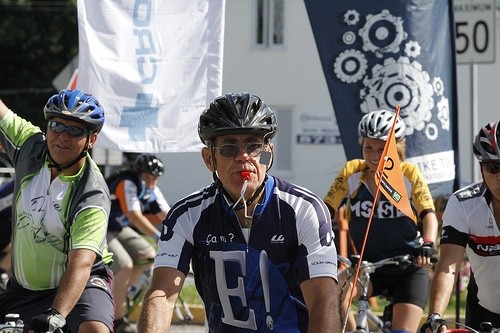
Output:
[210,137,270,158]
[481,159,500,174]
[152,169,161,178]
[48,119,89,138]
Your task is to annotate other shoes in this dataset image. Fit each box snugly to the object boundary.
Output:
[113,316,136,333]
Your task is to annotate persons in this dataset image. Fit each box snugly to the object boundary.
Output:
[136,93,342,333]
[323,110,438,333]
[0,88,114,333]
[104,154,170,333]
[420,120,500,333]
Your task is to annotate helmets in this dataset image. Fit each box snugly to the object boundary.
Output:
[359,110,406,143]
[42,88,104,133]
[473,120,500,161]
[198,93,278,145]
[135,154,164,176]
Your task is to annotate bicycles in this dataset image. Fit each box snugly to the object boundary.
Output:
[335,246,500,333]
[119,251,209,333]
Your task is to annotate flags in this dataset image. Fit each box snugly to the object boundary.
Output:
[303,0,458,198]
[77,0,226,153]
